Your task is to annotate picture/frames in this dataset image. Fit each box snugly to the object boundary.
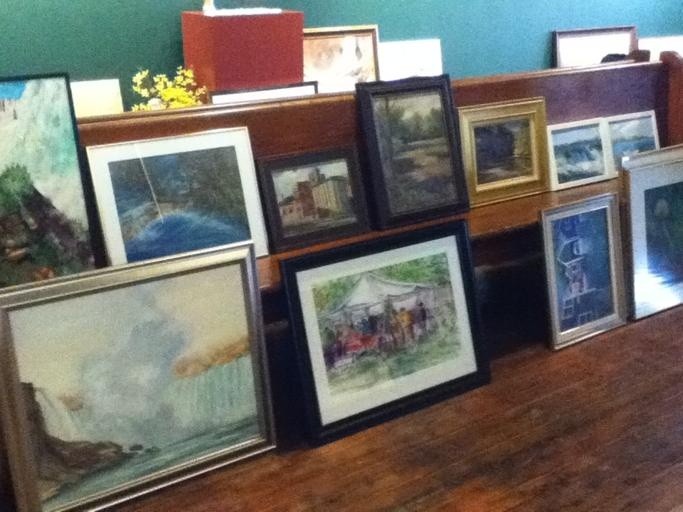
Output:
[1,71,127,287]
[546,117,611,192]
[0,241,284,512]
[602,108,661,181]
[550,25,641,69]
[378,37,444,81]
[536,190,633,354]
[253,142,371,253]
[82,124,272,268]
[354,71,470,232]
[456,95,548,210]
[209,81,320,106]
[277,215,494,452]
[619,141,682,322]
[304,24,382,94]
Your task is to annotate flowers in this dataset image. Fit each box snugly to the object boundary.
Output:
[131,66,206,113]
